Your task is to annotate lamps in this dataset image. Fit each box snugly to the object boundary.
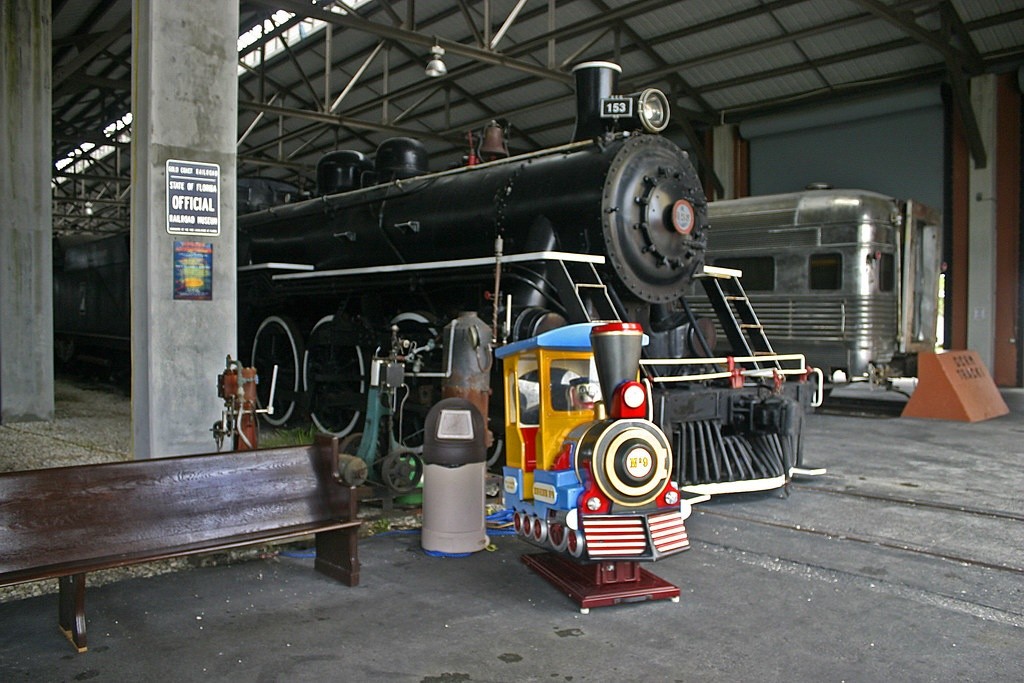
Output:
[425,46,447,77]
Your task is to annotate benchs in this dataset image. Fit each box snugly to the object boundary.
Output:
[0,431,364,652]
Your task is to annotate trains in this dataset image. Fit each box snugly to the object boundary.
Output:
[482,314,692,607]
[51,61,713,439]
[673,182,945,413]
